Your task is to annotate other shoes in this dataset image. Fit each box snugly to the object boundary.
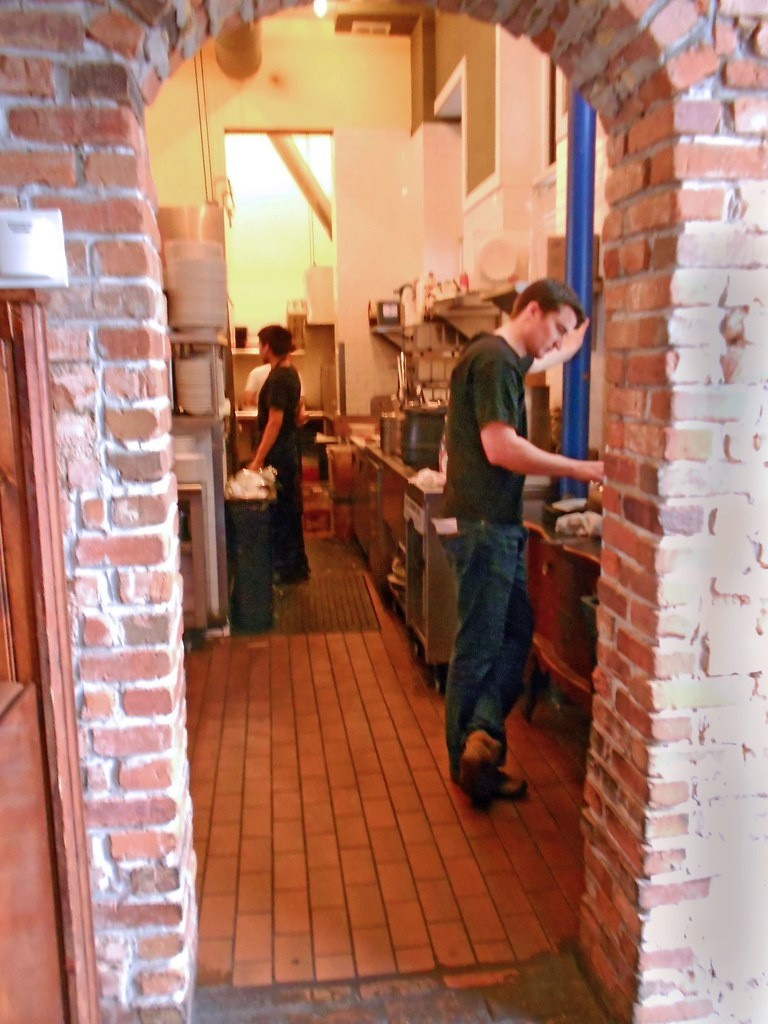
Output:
[447,770,527,797]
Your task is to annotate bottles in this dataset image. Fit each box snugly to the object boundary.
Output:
[424,271,437,321]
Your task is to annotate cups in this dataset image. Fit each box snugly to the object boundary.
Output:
[235,326,246,349]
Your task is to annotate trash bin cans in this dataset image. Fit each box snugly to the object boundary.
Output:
[225,499,273,630]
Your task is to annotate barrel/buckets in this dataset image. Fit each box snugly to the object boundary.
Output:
[326,443,354,499]
[403,408,445,465]
[330,491,354,543]
[381,413,406,456]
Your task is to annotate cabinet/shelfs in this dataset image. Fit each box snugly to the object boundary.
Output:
[352,444,378,555]
[233,349,323,420]
[177,483,213,631]
[523,517,601,725]
[172,335,227,428]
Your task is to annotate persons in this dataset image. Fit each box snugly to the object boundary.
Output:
[441,276,601,808]
[245,352,313,427]
[244,324,313,585]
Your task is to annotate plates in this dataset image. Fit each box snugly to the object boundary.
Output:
[165,240,227,334]
[174,433,206,485]
[175,358,224,416]
